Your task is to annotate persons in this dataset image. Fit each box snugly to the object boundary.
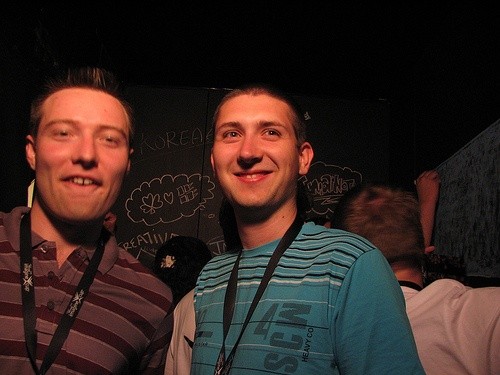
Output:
[0,67,175,375]
[414,170,439,249]
[345,185,500,375]
[191,81,425,375]
[155,236,212,308]
